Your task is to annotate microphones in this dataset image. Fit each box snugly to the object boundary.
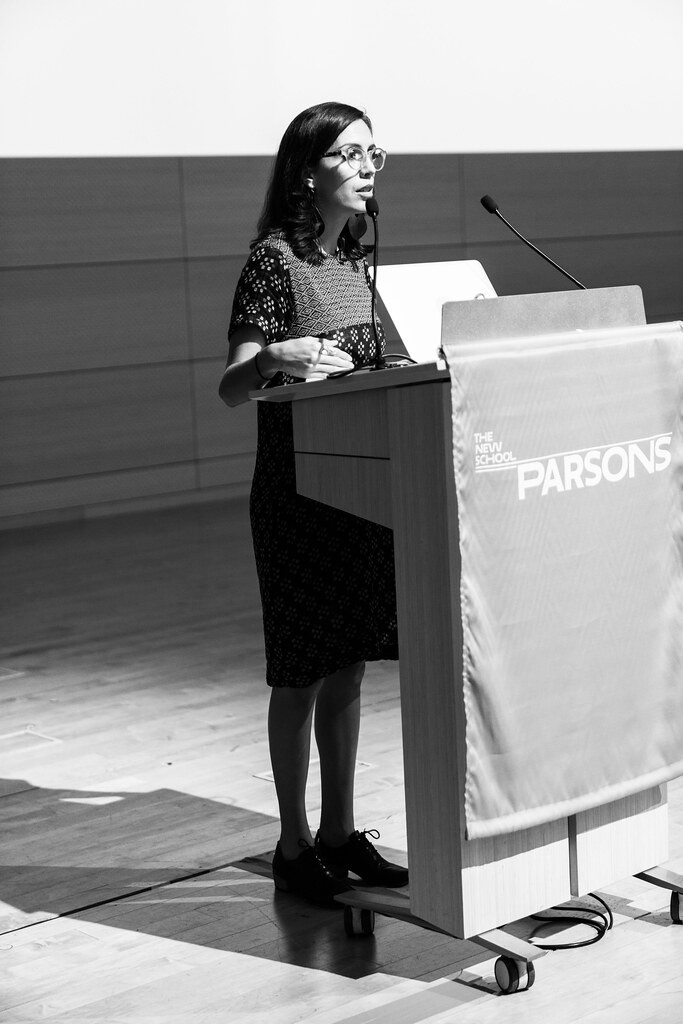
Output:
[480,195,587,289]
[327,198,395,380]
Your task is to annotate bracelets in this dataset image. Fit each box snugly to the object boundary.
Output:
[254,352,273,381]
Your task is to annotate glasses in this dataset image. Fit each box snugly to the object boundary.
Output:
[308,146,387,171]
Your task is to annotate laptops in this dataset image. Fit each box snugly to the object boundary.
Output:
[368,259,498,367]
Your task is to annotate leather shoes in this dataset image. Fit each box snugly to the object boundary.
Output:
[272,828,409,911]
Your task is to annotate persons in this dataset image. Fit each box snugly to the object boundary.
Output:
[218,99,409,912]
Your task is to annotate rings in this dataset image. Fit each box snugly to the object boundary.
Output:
[318,343,337,357]
